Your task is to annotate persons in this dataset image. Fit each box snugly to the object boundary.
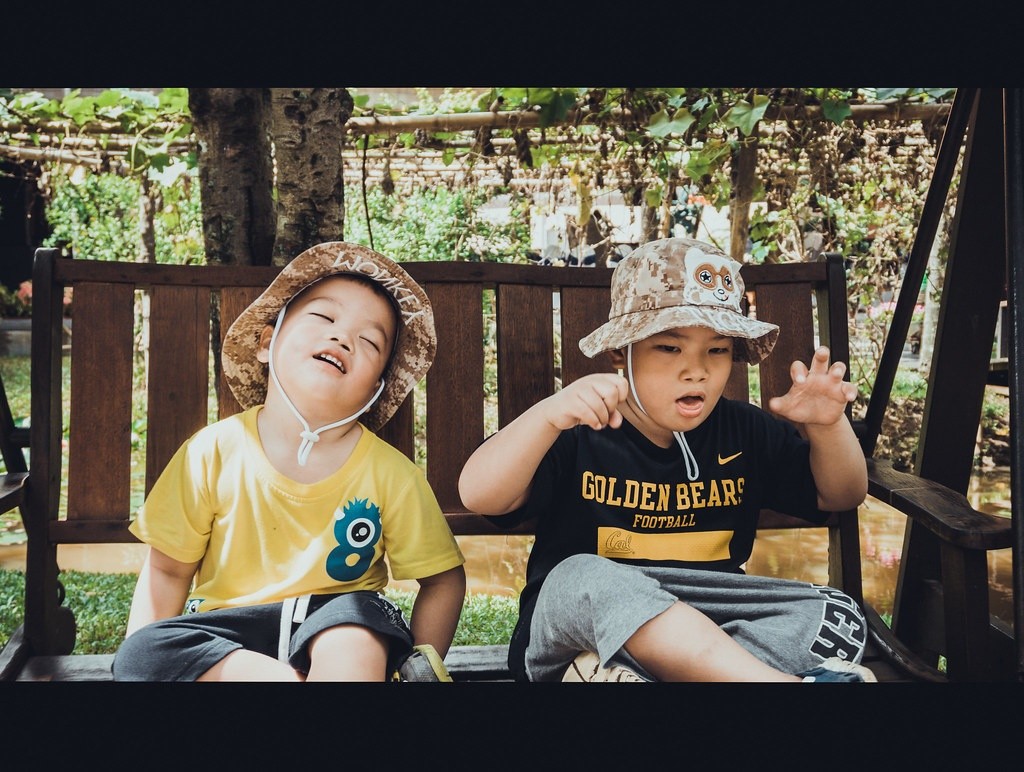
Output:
[110,240,466,682]
[459,237,877,683]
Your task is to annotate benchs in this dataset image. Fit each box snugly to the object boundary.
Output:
[0,245,1013,682]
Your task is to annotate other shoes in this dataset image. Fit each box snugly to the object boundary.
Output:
[392,644,456,682]
[561,650,647,682]
[795,656,878,682]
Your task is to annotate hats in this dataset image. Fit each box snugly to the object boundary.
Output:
[220,241,436,433]
[579,237,781,366]
